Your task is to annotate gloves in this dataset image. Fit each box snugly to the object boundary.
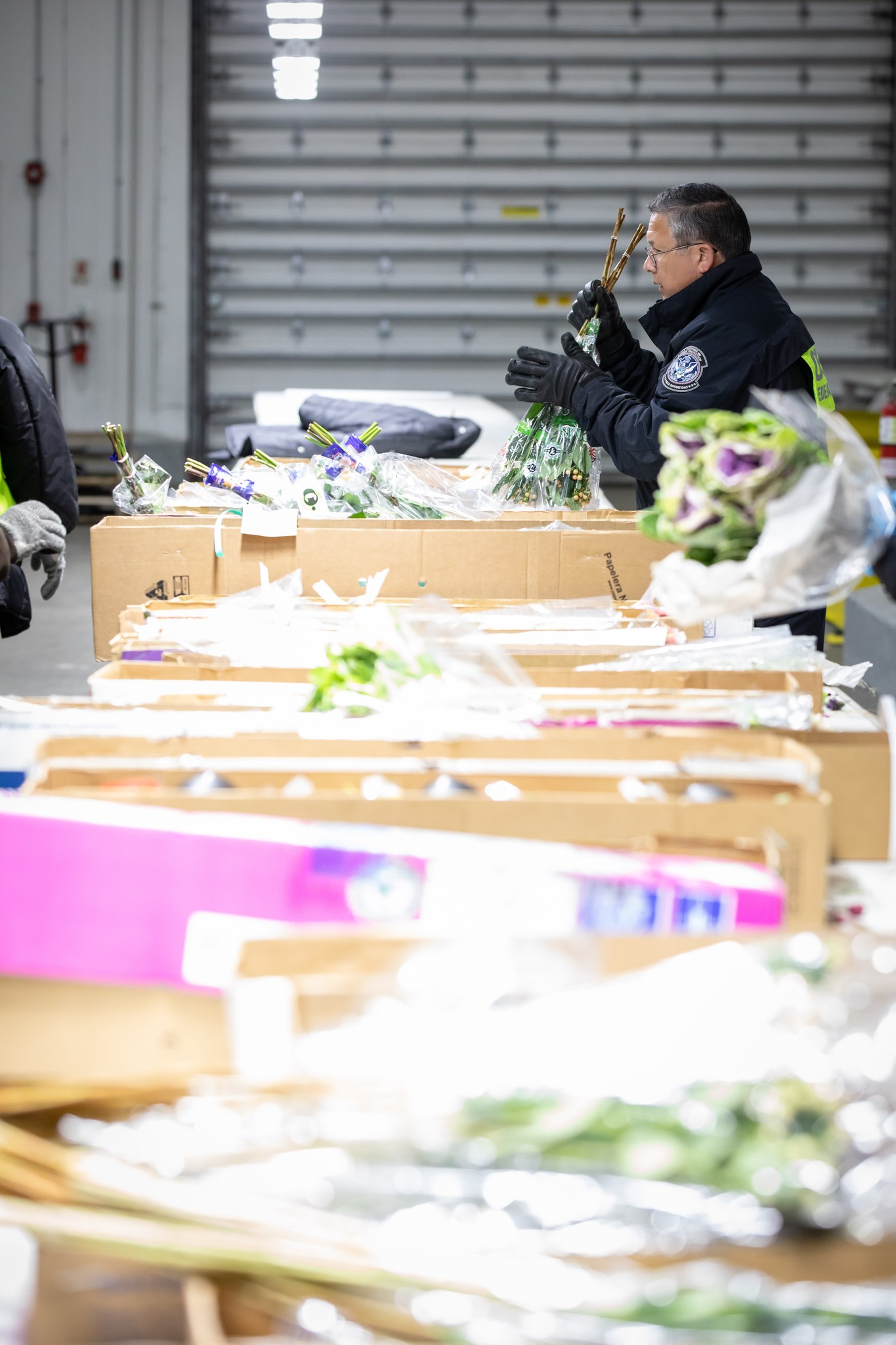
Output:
[566,279,630,354]
[504,331,602,415]
[31,550,67,601]
[0,499,67,563]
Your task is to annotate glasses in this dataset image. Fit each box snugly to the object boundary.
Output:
[645,243,717,267]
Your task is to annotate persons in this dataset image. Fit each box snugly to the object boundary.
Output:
[0,316,79,639]
[506,183,827,653]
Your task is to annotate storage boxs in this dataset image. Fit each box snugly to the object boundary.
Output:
[1,494,896,1345]
